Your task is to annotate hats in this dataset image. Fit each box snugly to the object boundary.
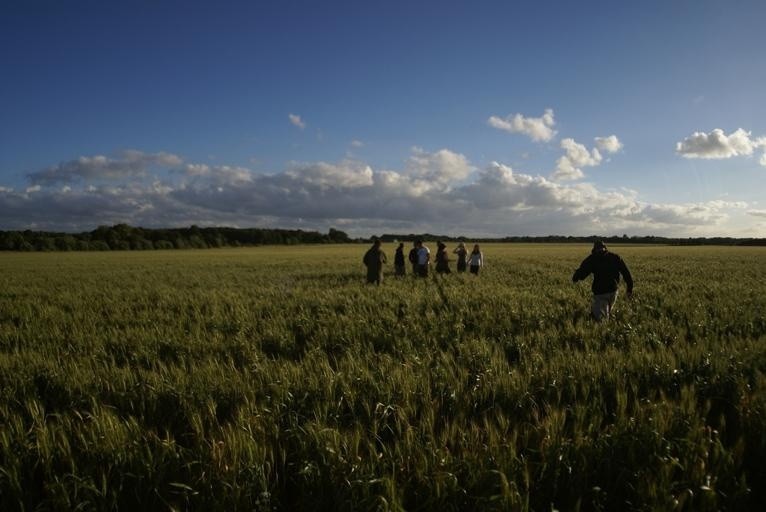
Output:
[458,241,466,248]
[591,239,607,252]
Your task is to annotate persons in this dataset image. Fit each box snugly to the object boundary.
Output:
[434,239,452,273]
[451,242,467,273]
[394,241,406,279]
[408,240,418,274]
[416,239,431,276]
[363,239,387,284]
[468,243,483,274]
[571,239,635,324]
